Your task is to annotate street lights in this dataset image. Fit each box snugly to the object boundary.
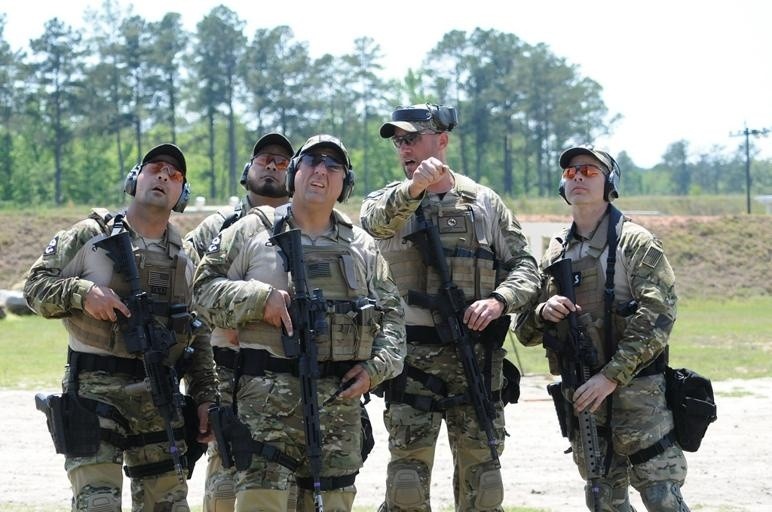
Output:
[728,128,770,215]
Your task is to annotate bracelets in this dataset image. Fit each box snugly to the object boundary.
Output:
[489,293,506,310]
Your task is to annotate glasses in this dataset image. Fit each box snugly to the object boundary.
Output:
[143,163,183,183]
[562,164,605,179]
[302,152,344,172]
[253,152,290,169]
[391,133,435,149]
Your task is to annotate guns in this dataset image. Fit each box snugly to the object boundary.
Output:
[543,257,605,512]
[91,233,191,484]
[402,225,498,462]
[265,228,329,512]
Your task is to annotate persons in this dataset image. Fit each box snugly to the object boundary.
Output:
[357,103,541,512]
[22,144,222,512]
[192,133,411,511]
[180,133,295,512]
[511,144,694,512]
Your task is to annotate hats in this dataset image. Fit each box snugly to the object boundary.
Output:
[142,143,187,176]
[254,133,294,156]
[559,147,612,171]
[380,104,438,138]
[299,135,350,168]
